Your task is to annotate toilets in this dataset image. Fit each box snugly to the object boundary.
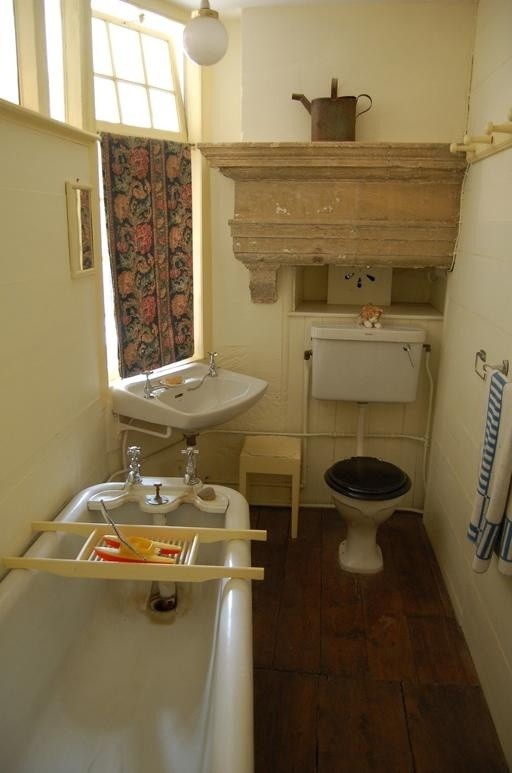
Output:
[308,326,428,574]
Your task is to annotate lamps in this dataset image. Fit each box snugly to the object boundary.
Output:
[181,0,229,67]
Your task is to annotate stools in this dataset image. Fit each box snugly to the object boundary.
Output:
[239,436,301,540]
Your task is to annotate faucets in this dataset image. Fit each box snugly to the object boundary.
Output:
[207,351,219,376]
[180,447,200,485]
[141,371,169,399]
[126,445,143,486]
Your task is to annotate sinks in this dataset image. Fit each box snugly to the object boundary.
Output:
[109,361,269,435]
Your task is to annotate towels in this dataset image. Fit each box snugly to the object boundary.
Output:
[467,369,512,577]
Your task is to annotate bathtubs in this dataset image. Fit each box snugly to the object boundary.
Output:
[0,476,255,773]
